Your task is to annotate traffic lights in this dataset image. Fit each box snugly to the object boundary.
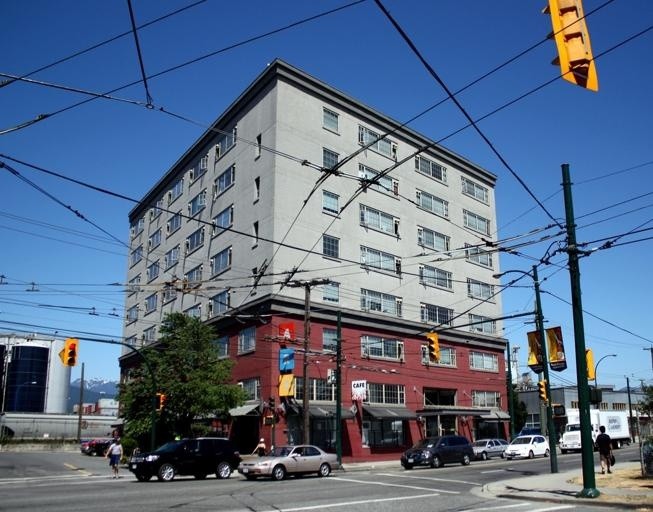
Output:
[426,330,440,362]
[537,378,548,401]
[154,392,166,411]
[62,338,78,366]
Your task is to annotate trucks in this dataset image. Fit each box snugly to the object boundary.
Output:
[559,407,630,455]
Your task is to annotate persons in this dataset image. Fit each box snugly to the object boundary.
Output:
[250,438,267,457]
[595,425,614,475]
[105,437,124,480]
[173,432,181,441]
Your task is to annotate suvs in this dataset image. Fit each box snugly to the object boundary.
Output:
[128,437,238,482]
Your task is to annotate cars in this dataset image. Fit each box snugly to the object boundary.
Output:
[401,435,474,468]
[82,439,112,457]
[503,433,550,460]
[472,438,508,460]
[237,444,338,480]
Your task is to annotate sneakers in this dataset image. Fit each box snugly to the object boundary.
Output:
[601,468,611,473]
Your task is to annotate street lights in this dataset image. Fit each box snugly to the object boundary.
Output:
[595,354,616,409]
[492,265,558,473]
[542,0,599,92]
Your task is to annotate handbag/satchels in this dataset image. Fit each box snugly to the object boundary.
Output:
[108,450,112,457]
[610,455,615,466]
